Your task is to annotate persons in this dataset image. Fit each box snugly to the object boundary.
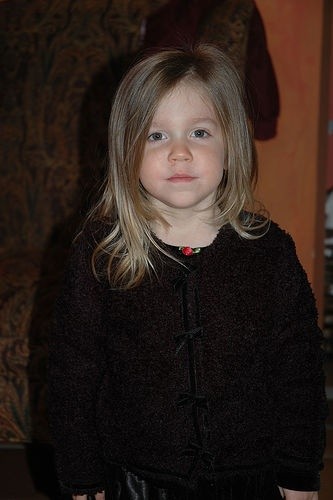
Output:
[49,49,328,500]
